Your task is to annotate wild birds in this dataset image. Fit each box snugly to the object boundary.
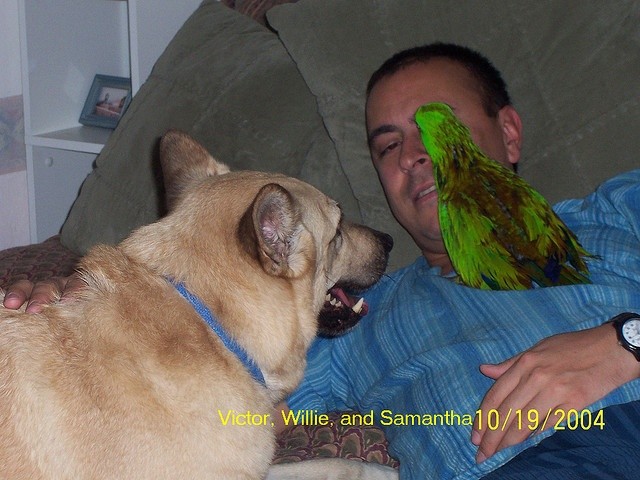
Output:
[416,102,605,291]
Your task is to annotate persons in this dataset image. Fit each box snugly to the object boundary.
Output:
[2,43,640,479]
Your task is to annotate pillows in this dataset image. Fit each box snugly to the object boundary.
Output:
[265,0,640,273]
[57,0,364,259]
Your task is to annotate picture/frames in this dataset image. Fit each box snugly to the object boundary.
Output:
[75,73,132,131]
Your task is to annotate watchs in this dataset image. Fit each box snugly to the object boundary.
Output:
[612,312,640,363]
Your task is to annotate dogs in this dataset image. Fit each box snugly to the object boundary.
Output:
[0,128,401,480]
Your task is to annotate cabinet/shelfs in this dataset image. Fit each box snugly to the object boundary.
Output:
[20,0,203,243]
